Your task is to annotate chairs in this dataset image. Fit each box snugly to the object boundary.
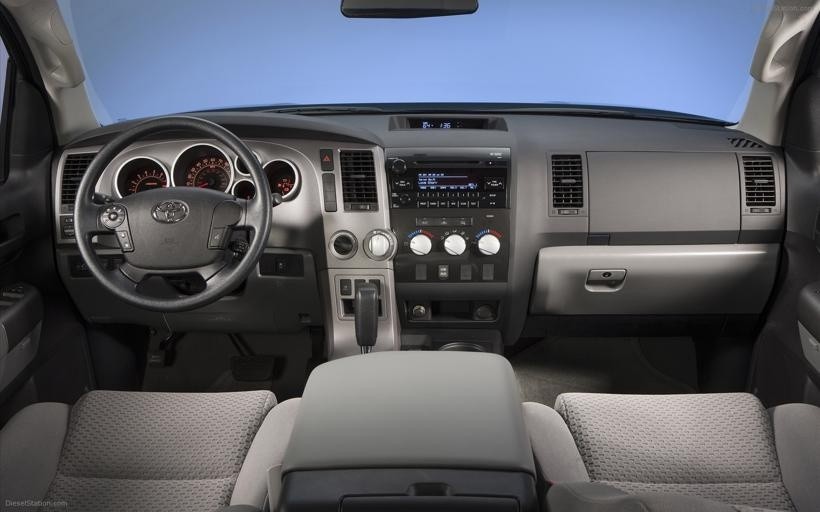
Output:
[521,392,820,512]
[0,388,303,512]
